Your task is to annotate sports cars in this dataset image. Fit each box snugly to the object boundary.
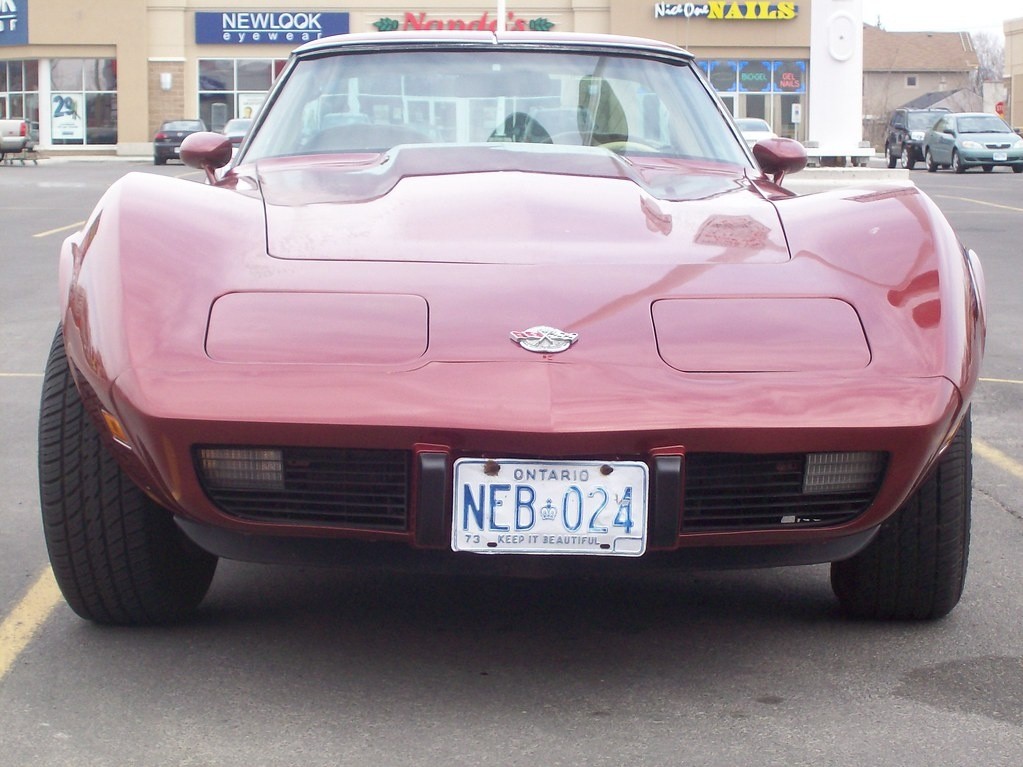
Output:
[36,28,989,626]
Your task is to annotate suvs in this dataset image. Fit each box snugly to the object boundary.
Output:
[885,107,954,171]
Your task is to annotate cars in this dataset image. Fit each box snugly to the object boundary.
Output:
[153,118,208,166]
[733,117,778,154]
[220,118,254,150]
[922,111,1023,174]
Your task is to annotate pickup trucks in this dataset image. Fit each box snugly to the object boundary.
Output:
[0,114,35,162]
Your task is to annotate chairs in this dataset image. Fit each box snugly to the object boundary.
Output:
[543,132,661,149]
[310,124,429,151]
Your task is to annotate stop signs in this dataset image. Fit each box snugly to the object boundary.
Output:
[994,102,1007,117]
